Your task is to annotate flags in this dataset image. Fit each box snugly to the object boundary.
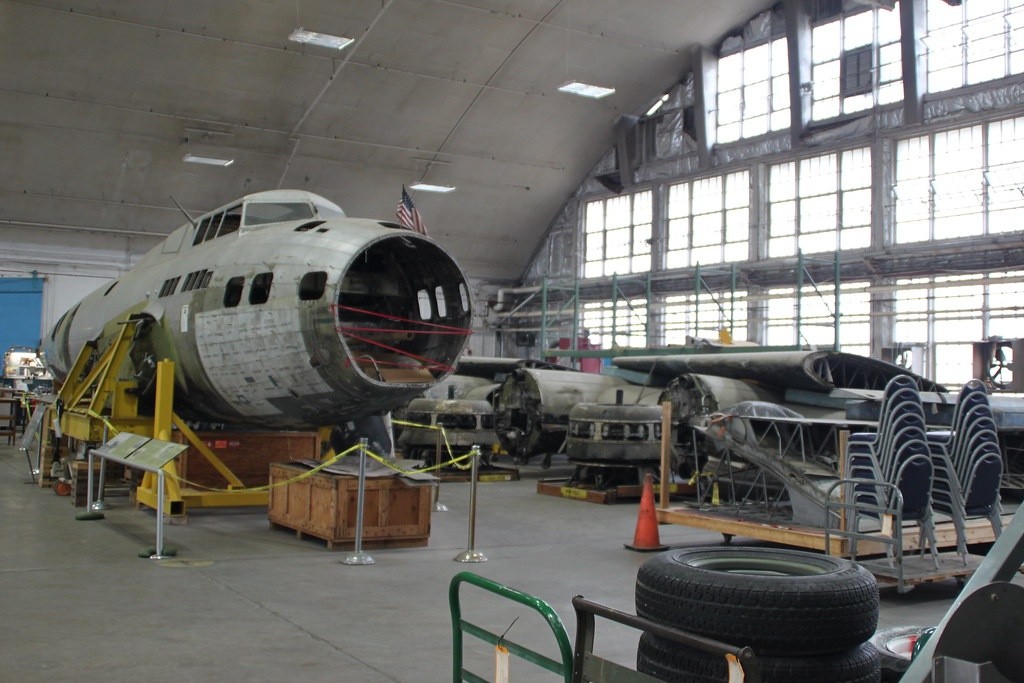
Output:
[395,184,428,237]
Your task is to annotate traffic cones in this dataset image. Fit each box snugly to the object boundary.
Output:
[623,473,670,553]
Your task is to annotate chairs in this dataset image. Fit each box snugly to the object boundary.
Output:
[845,374,940,571]
[918,378,1003,567]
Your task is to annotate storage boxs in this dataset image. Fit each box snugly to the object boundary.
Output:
[266,461,432,542]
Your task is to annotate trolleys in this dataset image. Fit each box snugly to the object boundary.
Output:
[824,478,985,597]
